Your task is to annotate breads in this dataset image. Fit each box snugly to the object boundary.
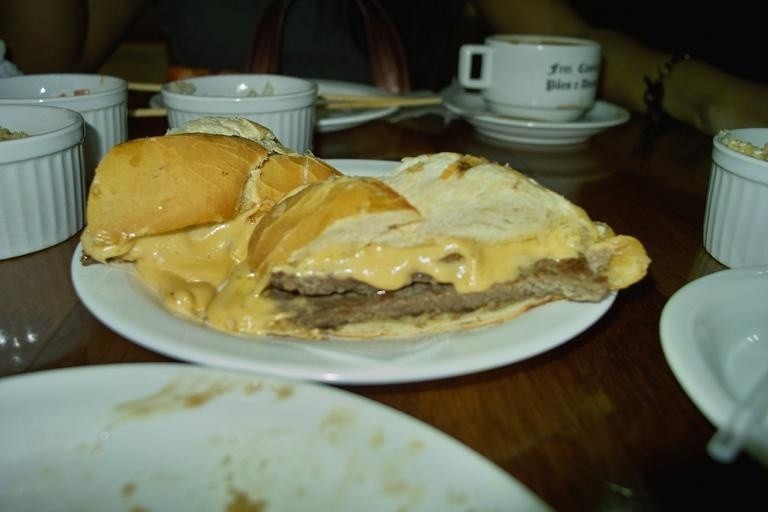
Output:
[86,132,423,278]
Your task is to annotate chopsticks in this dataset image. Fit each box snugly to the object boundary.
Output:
[128,81,442,118]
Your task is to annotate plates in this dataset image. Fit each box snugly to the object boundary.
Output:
[68,154,620,387]
[154,75,398,135]
[659,266,768,452]
[1,362,552,512]
[438,90,628,151]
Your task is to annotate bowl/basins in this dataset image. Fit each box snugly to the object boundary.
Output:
[704,124,768,269]
[0,70,130,261]
[163,72,319,149]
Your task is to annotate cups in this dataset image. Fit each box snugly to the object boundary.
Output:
[460,33,602,120]
